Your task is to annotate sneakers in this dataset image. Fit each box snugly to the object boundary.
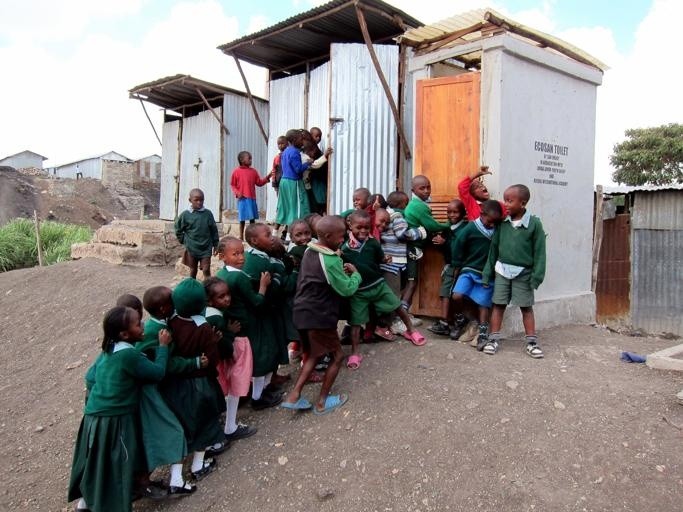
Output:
[427,319,450,335]
[525,341,544,358]
[303,374,322,383]
[408,313,422,326]
[449,314,466,339]
[458,321,478,342]
[469,326,479,347]
[476,322,488,351]
[138,479,170,501]
[347,352,364,371]
[374,325,395,342]
[313,354,332,372]
[360,328,374,342]
[272,375,289,384]
[482,338,499,355]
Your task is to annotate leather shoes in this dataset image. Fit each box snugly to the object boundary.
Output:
[190,455,217,479]
[167,479,197,499]
[249,394,283,410]
[264,383,286,393]
[223,421,258,442]
[204,441,232,457]
[78,497,91,512]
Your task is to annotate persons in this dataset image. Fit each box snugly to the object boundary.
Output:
[232,152,274,239]
[175,189,220,280]
[275,129,314,242]
[278,216,349,413]
[265,236,286,264]
[67,307,171,512]
[270,137,286,201]
[114,293,197,496]
[340,211,425,370]
[481,184,546,358]
[200,277,241,453]
[353,188,368,212]
[449,201,505,351]
[243,223,289,409]
[308,127,329,213]
[215,236,269,441]
[390,176,452,335]
[374,192,427,342]
[287,220,327,382]
[427,198,468,335]
[135,287,208,386]
[372,210,389,241]
[169,278,231,456]
[298,130,330,193]
[457,165,516,224]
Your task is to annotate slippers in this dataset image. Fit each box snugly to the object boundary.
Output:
[313,392,349,415]
[280,396,313,409]
[402,329,428,346]
